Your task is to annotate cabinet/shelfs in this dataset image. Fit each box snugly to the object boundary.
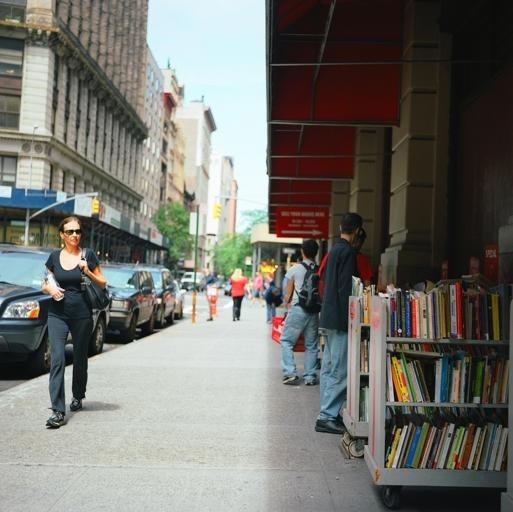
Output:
[340,273,370,458]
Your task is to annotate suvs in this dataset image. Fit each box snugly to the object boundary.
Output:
[97,261,157,346]
[182,272,207,292]
[0,239,113,371]
[122,262,177,330]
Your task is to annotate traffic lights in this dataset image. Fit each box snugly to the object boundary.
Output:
[212,202,222,221]
[91,198,99,215]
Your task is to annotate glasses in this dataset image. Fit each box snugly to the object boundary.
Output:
[62,229,83,235]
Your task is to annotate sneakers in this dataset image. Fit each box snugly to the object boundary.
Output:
[315,419,345,433]
[46,412,65,428]
[69,398,83,411]
[283,376,297,385]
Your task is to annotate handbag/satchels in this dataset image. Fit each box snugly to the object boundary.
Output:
[86,280,110,310]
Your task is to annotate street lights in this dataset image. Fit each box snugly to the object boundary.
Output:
[24,125,40,242]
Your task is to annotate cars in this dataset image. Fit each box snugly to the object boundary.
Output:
[172,279,186,320]
[213,280,231,296]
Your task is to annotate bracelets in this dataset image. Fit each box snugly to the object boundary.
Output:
[91,275,96,282]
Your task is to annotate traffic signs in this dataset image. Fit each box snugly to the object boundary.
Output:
[275,205,329,239]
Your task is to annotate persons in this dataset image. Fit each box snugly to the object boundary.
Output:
[261,288,284,323]
[280,238,319,386]
[441,262,448,280]
[249,271,263,307]
[282,274,291,309]
[318,228,372,300]
[315,212,363,434]
[263,272,275,289]
[203,268,218,321]
[42,216,106,429]
[228,268,251,321]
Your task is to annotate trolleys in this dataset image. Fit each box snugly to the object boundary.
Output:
[338,294,368,461]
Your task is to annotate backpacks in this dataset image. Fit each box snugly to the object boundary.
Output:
[292,261,322,314]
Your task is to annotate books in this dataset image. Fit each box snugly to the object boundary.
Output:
[351,276,509,472]
